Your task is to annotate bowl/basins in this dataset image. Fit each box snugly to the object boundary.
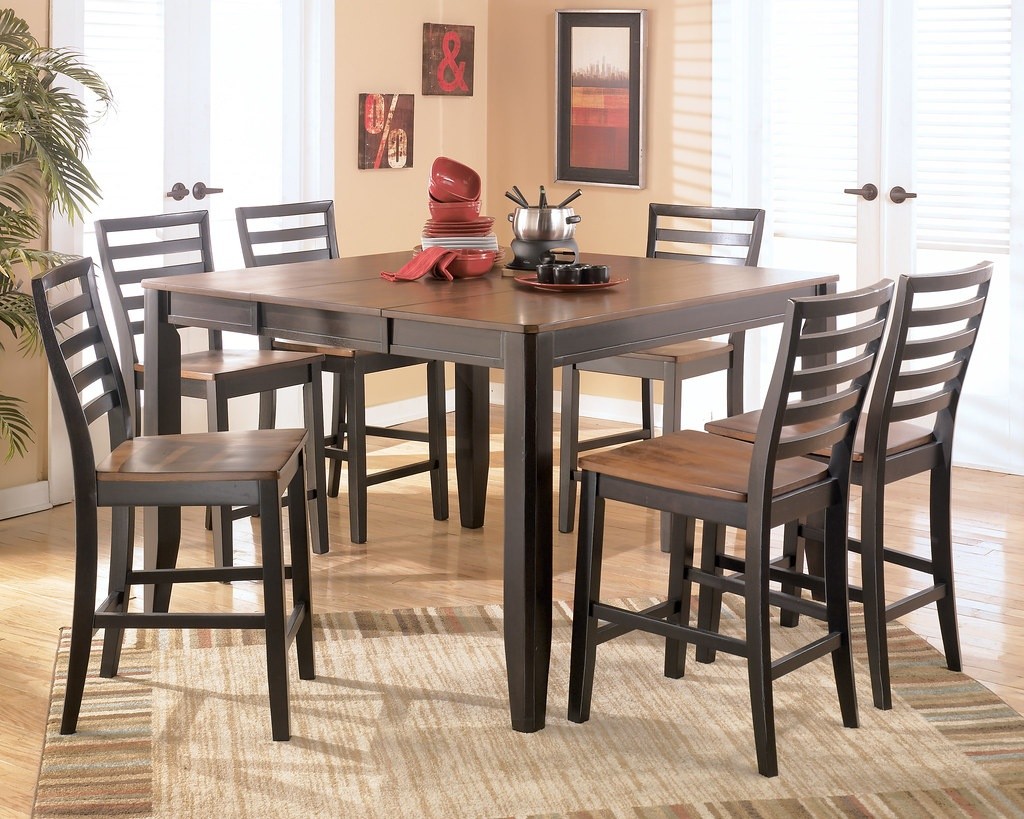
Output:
[426,155,483,221]
[445,249,496,277]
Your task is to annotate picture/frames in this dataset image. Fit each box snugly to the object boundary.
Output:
[555,10,649,190]
[422,23,474,97]
[359,93,414,170]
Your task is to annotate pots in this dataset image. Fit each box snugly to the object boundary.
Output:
[503,205,581,271]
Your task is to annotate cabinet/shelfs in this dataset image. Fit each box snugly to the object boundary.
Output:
[48,0,336,509]
[710,0,1024,478]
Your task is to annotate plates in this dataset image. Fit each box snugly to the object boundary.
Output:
[513,273,629,293]
[412,214,506,263]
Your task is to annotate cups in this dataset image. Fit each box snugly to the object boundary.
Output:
[583,264,610,283]
[571,263,590,284]
[536,264,561,284]
[553,266,581,284]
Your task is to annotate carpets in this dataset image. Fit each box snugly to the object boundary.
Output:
[31,593,1022,818]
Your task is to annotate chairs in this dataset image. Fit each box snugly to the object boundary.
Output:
[95,209,328,569]
[693,261,991,709]
[234,202,449,544]
[570,279,893,777]
[558,201,763,551]
[32,256,317,741]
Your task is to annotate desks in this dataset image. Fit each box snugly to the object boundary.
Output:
[140,251,840,733]
